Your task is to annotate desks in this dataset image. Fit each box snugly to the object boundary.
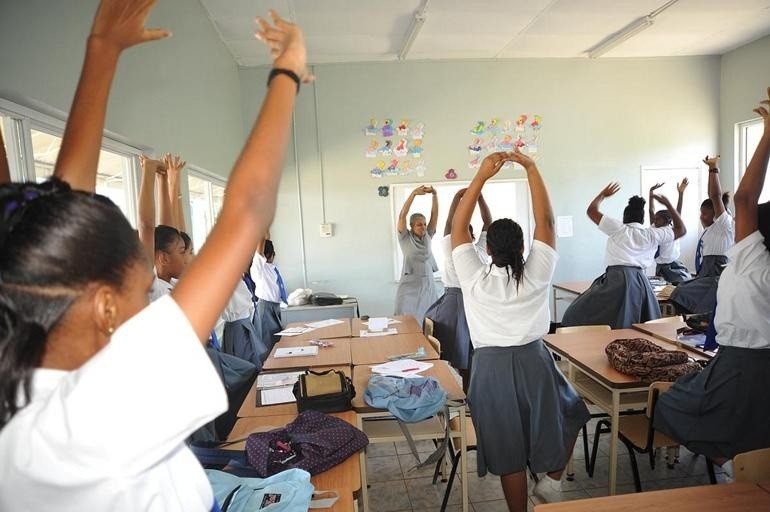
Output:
[534,481,770,512]
[552,280,678,324]
[633,314,718,358]
[537,328,708,496]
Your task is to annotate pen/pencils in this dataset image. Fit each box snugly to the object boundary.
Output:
[402,368,420,372]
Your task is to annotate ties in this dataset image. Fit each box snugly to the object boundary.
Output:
[703,296,719,351]
[654,245,661,259]
[274,267,289,306]
[210,329,220,350]
[694,230,708,272]
[244,275,259,316]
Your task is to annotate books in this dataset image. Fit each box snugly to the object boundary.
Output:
[273,345,318,357]
[255,370,307,390]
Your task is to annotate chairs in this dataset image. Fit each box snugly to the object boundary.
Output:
[555,325,611,473]
[643,314,701,459]
[588,380,718,493]
[733,448,770,482]
[424,316,433,336]
[432,415,539,512]
[425,334,441,359]
[644,314,685,324]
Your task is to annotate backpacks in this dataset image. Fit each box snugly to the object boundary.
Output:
[363,374,447,424]
[244,409,370,478]
[292,368,357,415]
[203,466,316,512]
[604,338,701,383]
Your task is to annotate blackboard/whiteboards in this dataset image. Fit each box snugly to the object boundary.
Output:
[389,179,533,284]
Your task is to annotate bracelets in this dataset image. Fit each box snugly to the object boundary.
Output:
[268,67,303,92]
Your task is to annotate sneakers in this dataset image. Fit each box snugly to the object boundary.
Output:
[533,477,566,503]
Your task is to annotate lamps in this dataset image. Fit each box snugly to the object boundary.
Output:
[587,17,654,59]
[396,11,426,61]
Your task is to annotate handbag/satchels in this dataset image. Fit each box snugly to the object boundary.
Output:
[310,292,344,306]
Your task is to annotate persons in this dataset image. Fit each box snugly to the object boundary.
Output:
[137,154,288,445]
[452,151,591,512]
[0,1,316,512]
[654,88,770,484]
[435,187,494,380]
[562,153,737,331]
[393,186,440,332]
